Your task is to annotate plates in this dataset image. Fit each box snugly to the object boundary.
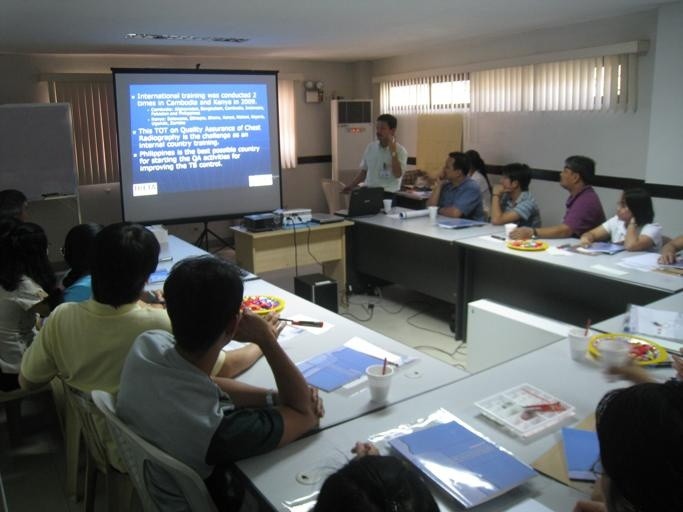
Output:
[240,295,285,314]
[588,333,670,366]
[508,239,549,250]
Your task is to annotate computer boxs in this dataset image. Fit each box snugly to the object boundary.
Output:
[294,273,337,314]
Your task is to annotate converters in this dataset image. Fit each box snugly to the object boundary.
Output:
[369,302,375,309]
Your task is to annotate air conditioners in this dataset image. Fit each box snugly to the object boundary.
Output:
[330,99,374,184]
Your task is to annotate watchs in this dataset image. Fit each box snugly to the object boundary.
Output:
[265,387,275,406]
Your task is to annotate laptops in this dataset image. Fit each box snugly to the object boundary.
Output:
[334,187,384,217]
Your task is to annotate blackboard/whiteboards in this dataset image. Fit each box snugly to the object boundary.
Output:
[0,103,78,202]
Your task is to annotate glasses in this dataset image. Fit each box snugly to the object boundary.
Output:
[57,246,70,257]
[561,164,583,176]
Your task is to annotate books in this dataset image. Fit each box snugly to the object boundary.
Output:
[369,407,537,511]
[622,302,681,352]
[474,380,575,443]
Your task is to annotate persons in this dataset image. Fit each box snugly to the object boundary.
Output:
[61,223,162,307]
[1,223,55,388]
[581,188,662,252]
[574,381,682,512]
[409,172,431,191]
[510,155,606,241]
[342,113,407,214]
[105,255,323,510]
[492,162,541,228]
[310,441,439,512]
[660,234,683,265]
[465,149,492,220]
[18,224,285,412]
[610,335,683,384]
[426,151,484,222]
[0,189,64,294]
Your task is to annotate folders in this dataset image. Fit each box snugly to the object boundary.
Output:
[561,428,606,481]
[437,219,486,229]
[388,420,538,509]
[295,346,389,392]
[576,242,626,255]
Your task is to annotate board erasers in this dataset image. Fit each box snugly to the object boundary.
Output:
[41,193,60,198]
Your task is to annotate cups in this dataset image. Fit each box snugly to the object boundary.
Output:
[383,199,392,212]
[504,223,517,238]
[598,341,629,382]
[366,365,393,404]
[567,328,592,362]
[427,206,438,220]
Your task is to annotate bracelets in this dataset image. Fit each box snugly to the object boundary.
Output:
[531,228,539,240]
[391,152,397,157]
[492,193,501,197]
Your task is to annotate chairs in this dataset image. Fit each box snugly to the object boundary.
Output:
[57,372,136,512]
[0,376,66,449]
[91,390,218,512]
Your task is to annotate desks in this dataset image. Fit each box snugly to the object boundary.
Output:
[456,227,683,342]
[142,235,256,295]
[236,327,683,512]
[589,290,683,356]
[230,220,356,308]
[223,278,472,454]
[335,207,519,340]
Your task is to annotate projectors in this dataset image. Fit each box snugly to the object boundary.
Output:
[273,208,312,229]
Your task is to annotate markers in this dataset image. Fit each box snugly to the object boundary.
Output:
[292,321,323,327]
[351,445,371,453]
[158,257,173,262]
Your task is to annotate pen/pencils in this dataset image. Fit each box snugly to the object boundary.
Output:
[492,235,506,240]
[263,316,293,321]
[558,244,570,249]
